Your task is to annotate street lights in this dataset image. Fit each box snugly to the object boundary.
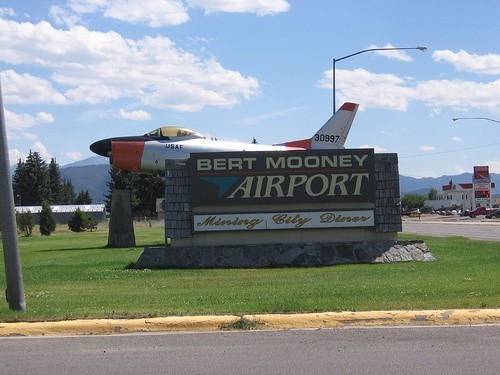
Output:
[17,195,21,206]
[333,46,430,116]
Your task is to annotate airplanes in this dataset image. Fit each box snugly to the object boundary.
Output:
[88,102,360,177]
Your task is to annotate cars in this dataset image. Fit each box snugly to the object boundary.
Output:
[401,208,421,216]
[485,208,500,219]
[430,209,469,215]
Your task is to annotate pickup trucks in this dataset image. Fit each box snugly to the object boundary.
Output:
[464,206,498,218]
